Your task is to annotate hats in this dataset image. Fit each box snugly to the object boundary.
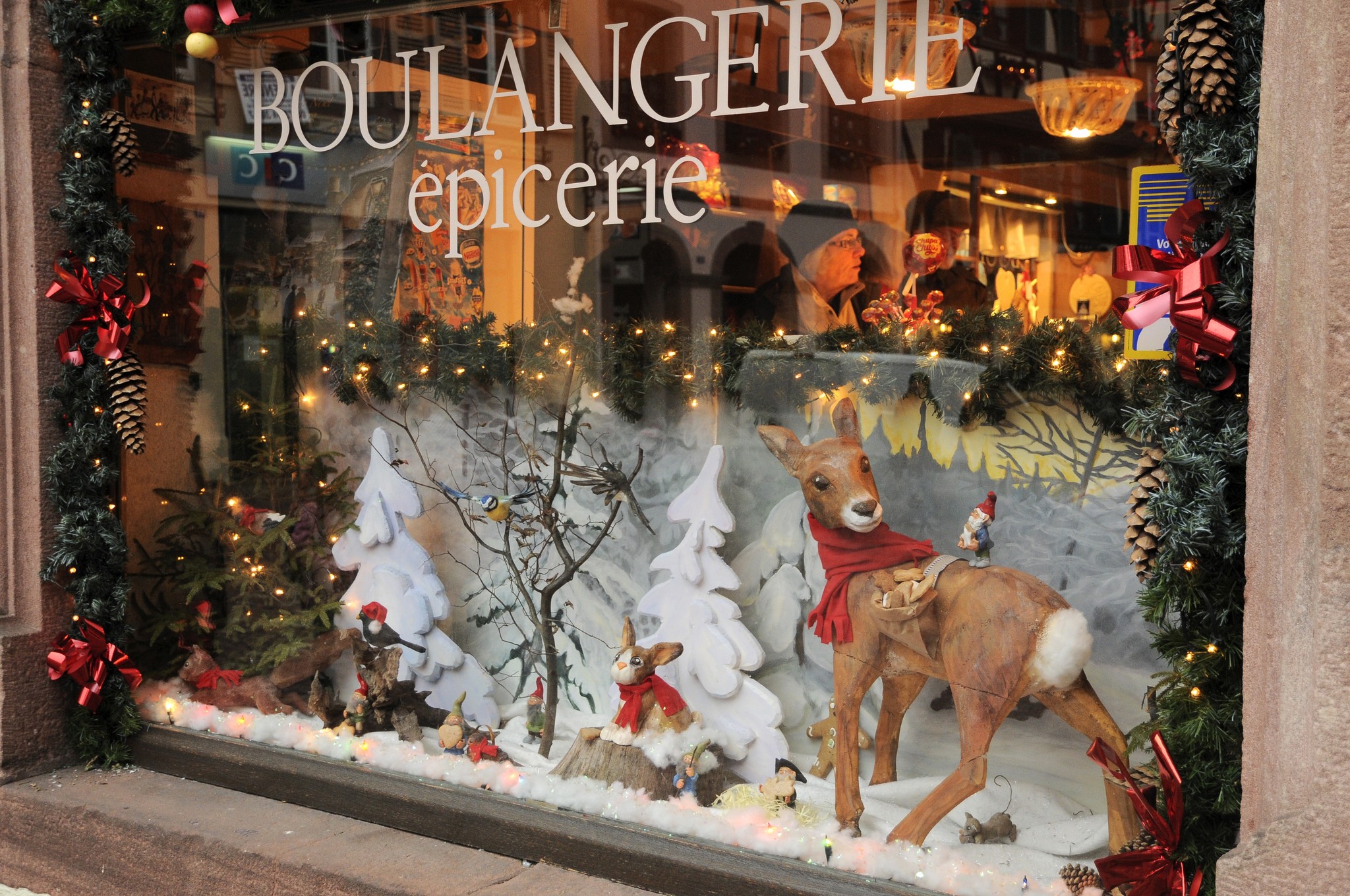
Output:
[778,199,860,267]
[906,190,971,231]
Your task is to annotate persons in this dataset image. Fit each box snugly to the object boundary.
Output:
[724,198,886,352]
[846,218,910,337]
[899,190,992,318]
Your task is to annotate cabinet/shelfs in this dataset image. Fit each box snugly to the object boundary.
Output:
[594,74,896,335]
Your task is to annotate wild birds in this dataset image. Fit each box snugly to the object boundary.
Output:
[553,457,657,538]
[357,601,427,653]
[435,478,546,525]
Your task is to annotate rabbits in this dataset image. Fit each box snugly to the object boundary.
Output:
[599,616,704,745]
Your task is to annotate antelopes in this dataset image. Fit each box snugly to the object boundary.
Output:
[758,397,1139,859]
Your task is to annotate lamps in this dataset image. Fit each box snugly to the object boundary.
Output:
[1020,72,1144,141]
[838,10,977,92]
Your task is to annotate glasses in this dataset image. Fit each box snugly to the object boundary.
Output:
[827,237,861,250]
